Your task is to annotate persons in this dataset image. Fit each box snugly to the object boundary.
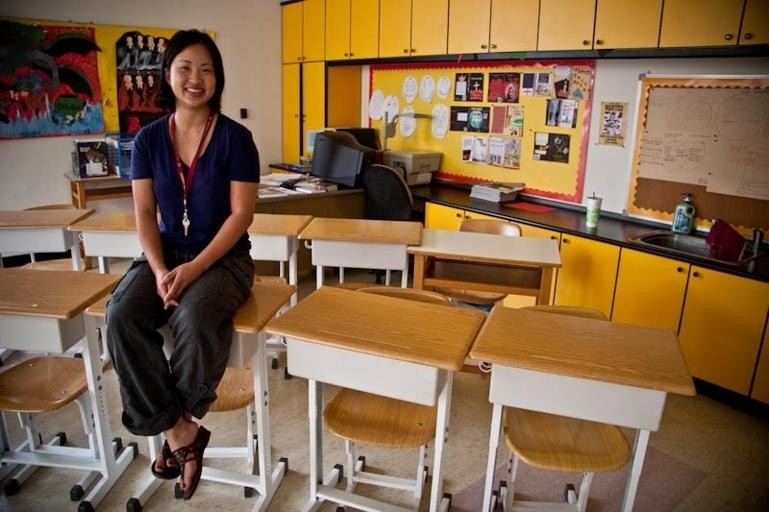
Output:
[102,27,259,503]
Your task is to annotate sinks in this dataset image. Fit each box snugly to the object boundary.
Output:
[629,230,753,266]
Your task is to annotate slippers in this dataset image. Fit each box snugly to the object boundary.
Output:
[151,439,181,479]
[170,424,210,500]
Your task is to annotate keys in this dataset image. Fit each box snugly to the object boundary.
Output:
[181,218,190,236]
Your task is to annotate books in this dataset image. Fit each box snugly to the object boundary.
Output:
[255,171,337,199]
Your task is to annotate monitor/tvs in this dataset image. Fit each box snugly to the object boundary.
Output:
[311,130,376,186]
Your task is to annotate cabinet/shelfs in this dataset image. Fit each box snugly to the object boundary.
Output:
[748,278,768,405]
[282,61,361,165]
[609,247,768,398]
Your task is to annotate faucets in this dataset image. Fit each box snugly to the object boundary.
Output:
[712,219,764,257]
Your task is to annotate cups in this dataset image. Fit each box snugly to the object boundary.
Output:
[586,197,603,229]
